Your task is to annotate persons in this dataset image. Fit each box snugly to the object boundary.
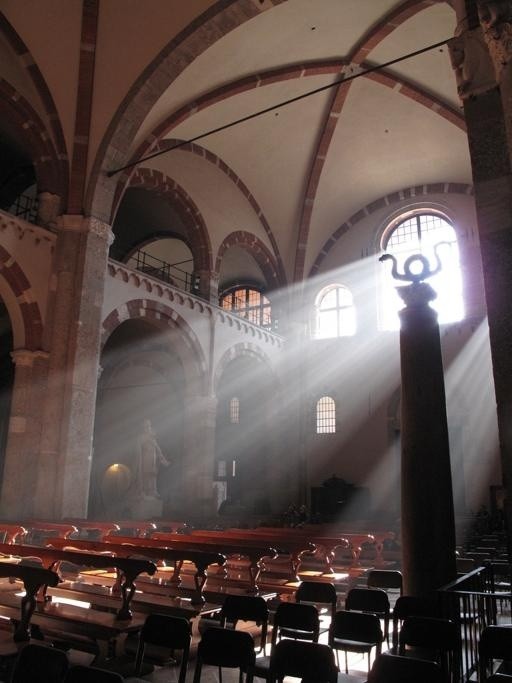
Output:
[295,504,311,528]
[135,416,170,501]
[281,502,299,528]
